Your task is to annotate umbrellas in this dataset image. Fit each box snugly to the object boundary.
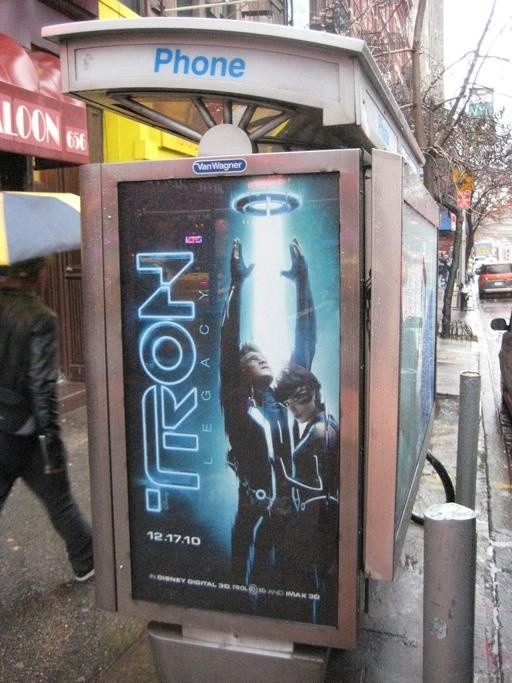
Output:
[0,191,80,267]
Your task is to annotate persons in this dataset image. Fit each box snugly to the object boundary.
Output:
[0,257,95,583]
[269,362,338,623]
[219,237,316,612]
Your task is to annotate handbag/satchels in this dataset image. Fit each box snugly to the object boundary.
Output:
[0,386,36,437]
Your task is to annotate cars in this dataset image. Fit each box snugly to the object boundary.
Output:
[491,310,512,419]
[473,255,512,299]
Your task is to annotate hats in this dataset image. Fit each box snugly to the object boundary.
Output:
[4,257,51,279]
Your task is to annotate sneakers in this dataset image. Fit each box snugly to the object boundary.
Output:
[72,557,95,582]
